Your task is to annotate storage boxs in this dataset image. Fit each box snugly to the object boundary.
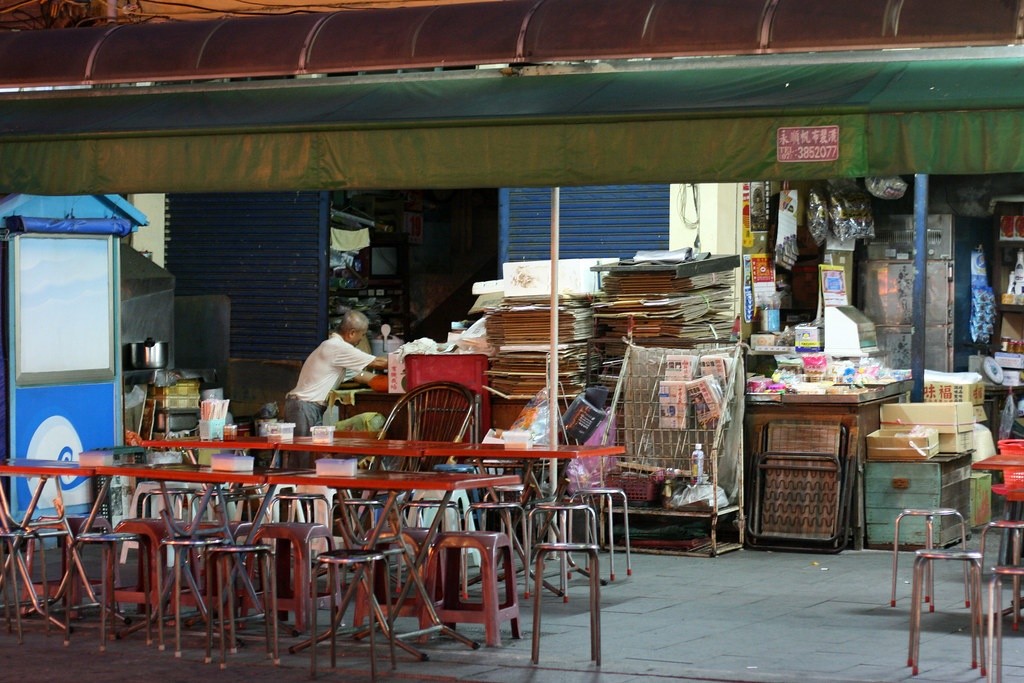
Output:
[867,426,939,460]
[751,331,781,349]
[795,327,825,352]
[973,405,987,422]
[905,381,984,405]
[880,402,974,453]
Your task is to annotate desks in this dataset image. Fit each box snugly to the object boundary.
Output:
[94,462,316,647]
[140,435,309,613]
[422,443,627,598]
[971,453,1024,630]
[0,457,155,635]
[268,472,522,660]
[276,437,473,594]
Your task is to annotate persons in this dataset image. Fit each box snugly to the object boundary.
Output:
[283,311,388,470]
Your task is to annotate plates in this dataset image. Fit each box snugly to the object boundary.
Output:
[983,356,1004,385]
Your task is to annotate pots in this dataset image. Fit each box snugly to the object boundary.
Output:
[370,334,404,372]
[129,336,169,368]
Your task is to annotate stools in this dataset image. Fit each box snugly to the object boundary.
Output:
[907,549,987,678]
[979,520,1024,631]
[0,488,632,683]
[890,507,970,614]
[987,566,1024,683]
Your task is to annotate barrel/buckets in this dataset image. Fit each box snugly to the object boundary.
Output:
[237,425,252,436]
[254,419,278,437]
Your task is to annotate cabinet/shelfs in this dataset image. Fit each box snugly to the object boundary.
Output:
[600,344,745,558]
[991,201,1024,390]
[863,449,976,551]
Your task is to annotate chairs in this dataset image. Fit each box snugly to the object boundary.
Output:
[366,381,475,476]
[745,415,861,555]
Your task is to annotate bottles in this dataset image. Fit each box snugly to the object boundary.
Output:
[662,479,672,510]
[691,443,704,485]
[328,270,359,288]
[1007,248,1024,293]
[224,424,237,440]
